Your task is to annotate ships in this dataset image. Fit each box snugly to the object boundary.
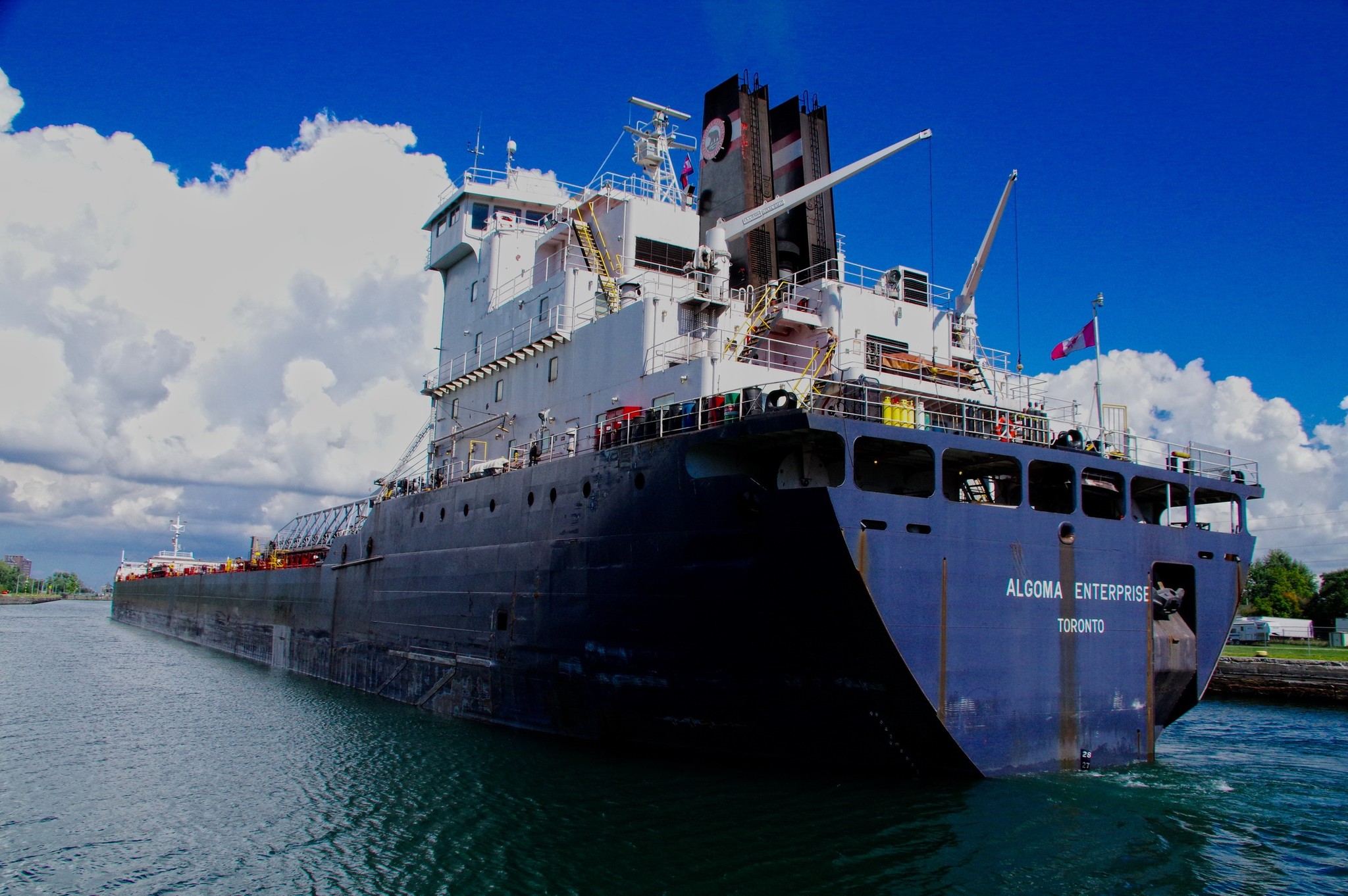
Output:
[113,68,1266,791]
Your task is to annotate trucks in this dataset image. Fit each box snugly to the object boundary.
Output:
[1225,621,1271,645]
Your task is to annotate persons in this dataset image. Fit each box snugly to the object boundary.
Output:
[820,326,838,376]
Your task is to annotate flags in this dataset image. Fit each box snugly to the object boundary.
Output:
[680,153,694,190]
[1051,319,1095,362]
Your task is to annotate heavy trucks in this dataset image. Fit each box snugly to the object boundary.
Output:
[1230,615,1316,640]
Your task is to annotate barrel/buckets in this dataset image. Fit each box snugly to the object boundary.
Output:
[593,387,762,453]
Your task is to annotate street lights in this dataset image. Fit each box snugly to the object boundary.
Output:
[14,574,106,598]
